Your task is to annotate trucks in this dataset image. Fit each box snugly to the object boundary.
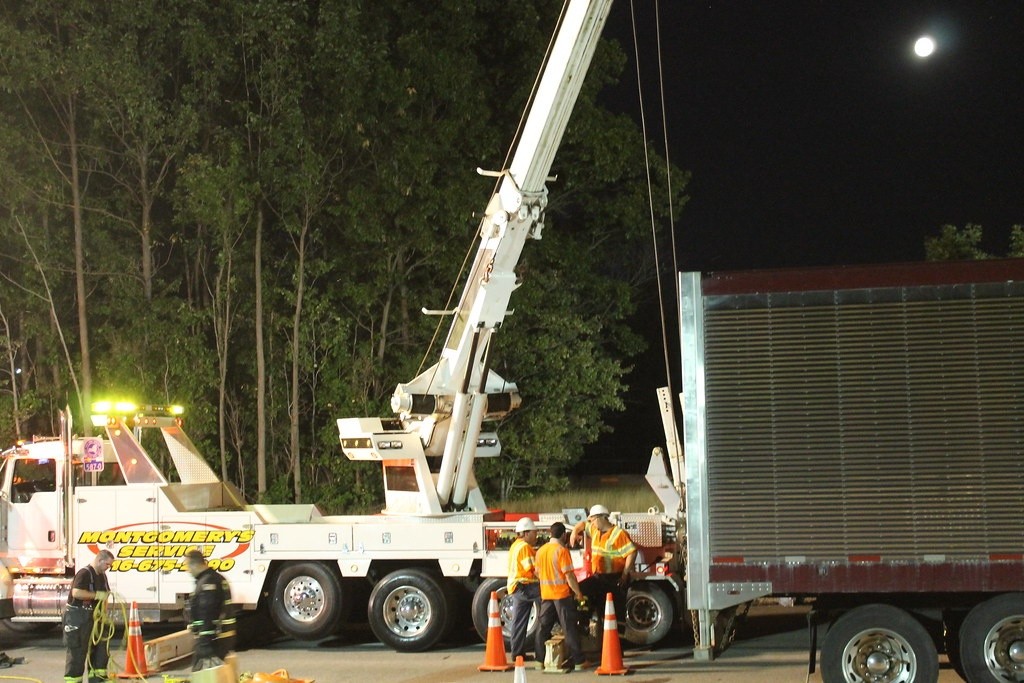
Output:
[676,269,1024,682]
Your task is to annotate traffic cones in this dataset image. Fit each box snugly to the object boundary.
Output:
[594,593,630,676]
[476,592,515,670]
[513,656,528,683]
[116,601,153,677]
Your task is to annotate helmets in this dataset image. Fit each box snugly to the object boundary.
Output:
[516,517,537,533]
[589,504,609,517]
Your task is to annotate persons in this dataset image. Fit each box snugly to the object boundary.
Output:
[61,549,117,683]
[506,504,638,672]
[181,549,237,674]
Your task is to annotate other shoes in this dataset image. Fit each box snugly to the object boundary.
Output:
[533,661,544,670]
[574,659,593,671]
[513,654,534,661]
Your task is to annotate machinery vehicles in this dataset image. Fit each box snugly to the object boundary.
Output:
[0,1,702,654]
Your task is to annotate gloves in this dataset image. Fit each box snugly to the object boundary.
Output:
[94,590,108,601]
